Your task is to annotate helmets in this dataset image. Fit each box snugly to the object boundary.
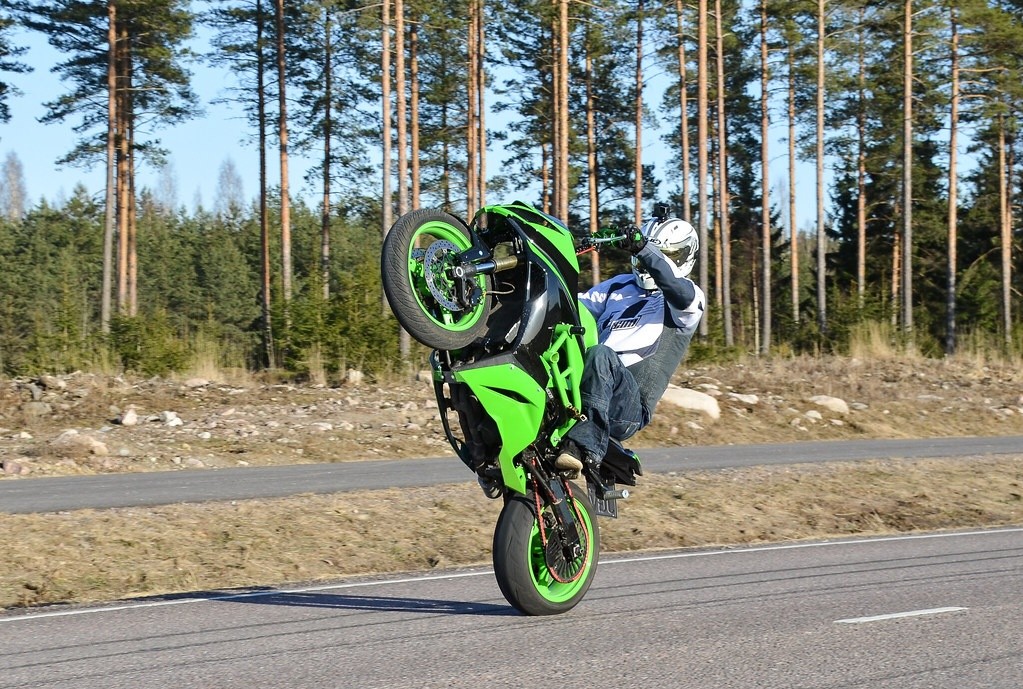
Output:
[630,216,700,290]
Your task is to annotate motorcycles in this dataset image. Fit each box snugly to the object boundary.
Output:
[380,200,668,616]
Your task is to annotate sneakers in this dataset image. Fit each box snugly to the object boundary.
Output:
[553,436,584,481]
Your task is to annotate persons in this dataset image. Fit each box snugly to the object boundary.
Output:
[477,216,704,498]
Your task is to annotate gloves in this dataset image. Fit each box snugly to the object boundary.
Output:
[617,223,649,254]
